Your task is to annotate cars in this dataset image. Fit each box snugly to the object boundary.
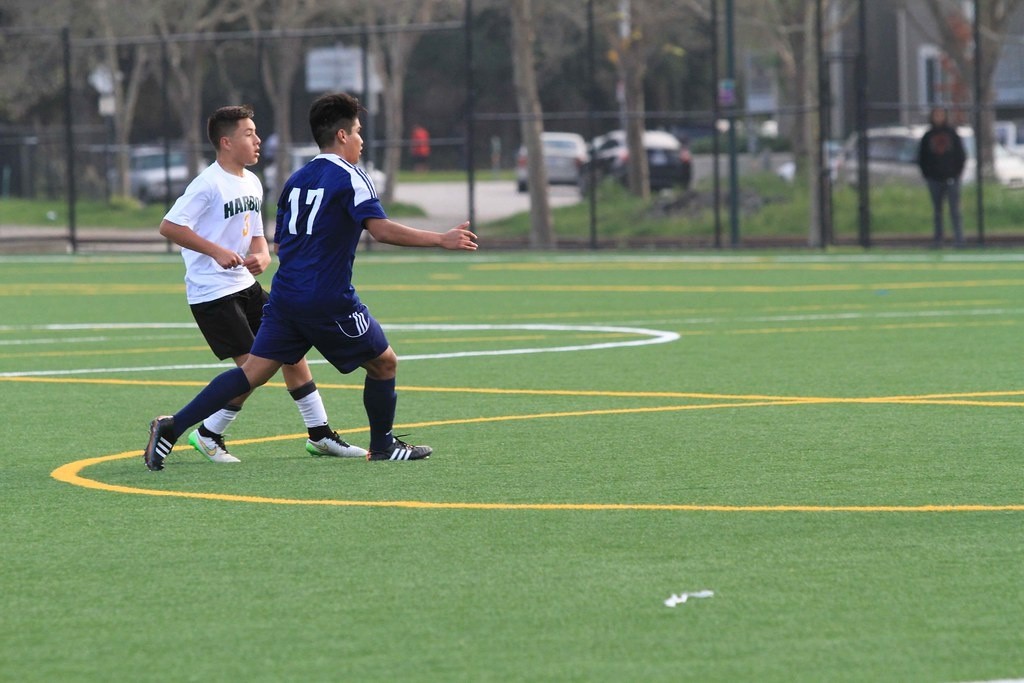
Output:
[578,129,693,196]
[122,143,193,204]
[252,134,389,206]
[514,131,592,193]
[775,111,1024,199]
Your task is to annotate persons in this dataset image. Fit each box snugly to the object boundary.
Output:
[143,91,479,471]
[409,124,430,169]
[917,109,968,251]
[160,105,369,465]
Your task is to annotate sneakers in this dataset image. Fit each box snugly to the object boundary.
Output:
[142,416,178,471]
[306,431,368,457]
[368,434,432,460]
[187,429,240,462]
